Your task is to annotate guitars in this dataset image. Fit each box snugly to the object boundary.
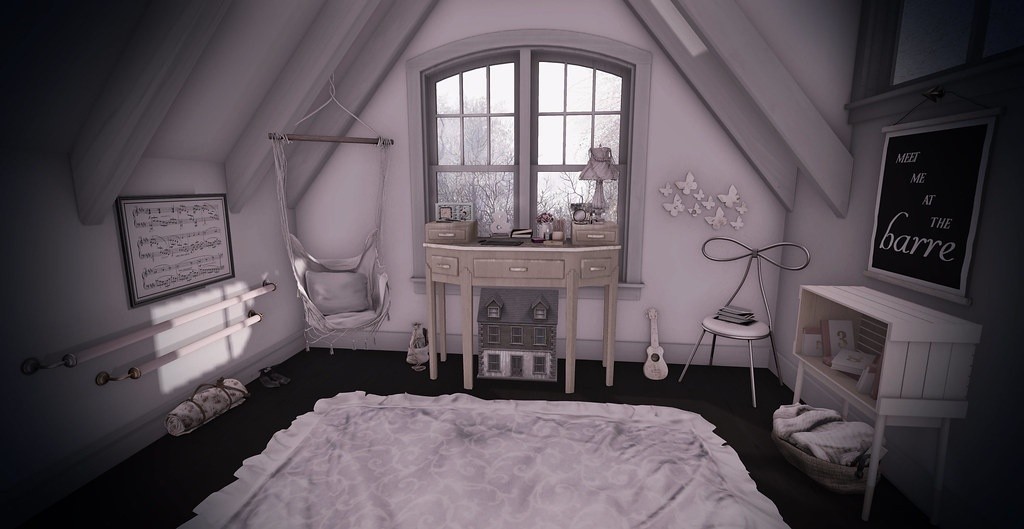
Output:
[643,308,668,381]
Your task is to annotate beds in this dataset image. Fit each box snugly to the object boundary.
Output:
[178,390,792,529]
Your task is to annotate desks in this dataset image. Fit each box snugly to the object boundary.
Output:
[422,236,622,394]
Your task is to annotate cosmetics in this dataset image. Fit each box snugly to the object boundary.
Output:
[586,210,606,222]
[553,230,564,241]
[544,229,552,241]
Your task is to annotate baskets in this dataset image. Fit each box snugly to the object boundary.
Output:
[769,429,877,496]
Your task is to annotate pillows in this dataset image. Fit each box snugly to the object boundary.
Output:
[304,269,370,316]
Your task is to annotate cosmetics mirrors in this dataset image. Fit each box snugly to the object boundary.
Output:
[573,209,586,222]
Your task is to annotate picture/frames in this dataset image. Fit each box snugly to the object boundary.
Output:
[116,193,235,308]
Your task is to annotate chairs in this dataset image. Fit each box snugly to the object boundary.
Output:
[678,236,811,408]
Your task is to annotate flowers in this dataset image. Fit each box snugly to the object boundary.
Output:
[535,212,554,225]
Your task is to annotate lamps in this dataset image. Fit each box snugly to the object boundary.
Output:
[577,143,621,224]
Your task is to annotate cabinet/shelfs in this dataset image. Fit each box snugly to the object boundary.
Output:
[792,283,984,529]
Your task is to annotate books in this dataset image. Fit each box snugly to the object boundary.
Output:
[802,327,823,355]
[856,363,876,394]
[822,318,855,355]
[830,347,876,375]
[512,229,532,238]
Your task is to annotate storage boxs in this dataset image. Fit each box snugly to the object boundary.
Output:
[772,436,881,494]
[571,220,619,245]
[425,220,477,244]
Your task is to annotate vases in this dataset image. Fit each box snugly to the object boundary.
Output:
[539,222,552,238]
